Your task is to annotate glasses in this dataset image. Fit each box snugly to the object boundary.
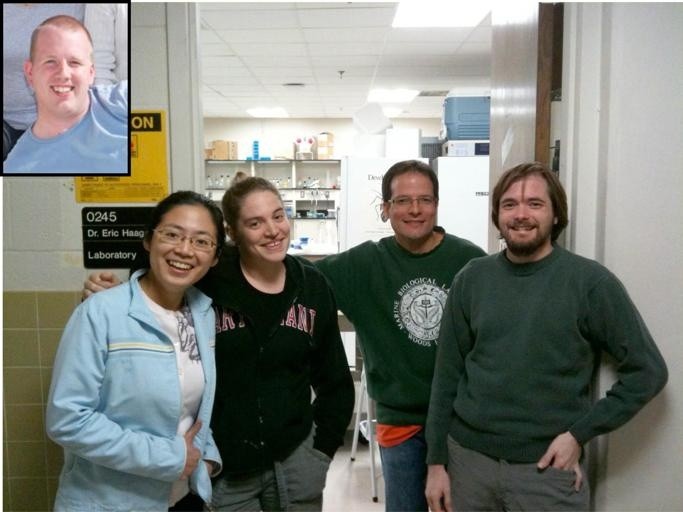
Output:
[387,195,436,206]
[152,228,216,253]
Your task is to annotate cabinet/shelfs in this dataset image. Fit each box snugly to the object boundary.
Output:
[204,159,347,257]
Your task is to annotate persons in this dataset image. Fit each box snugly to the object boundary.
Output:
[42,187,226,512]
[421,159,670,512]
[3,10,131,173]
[310,156,495,512]
[80,175,355,511]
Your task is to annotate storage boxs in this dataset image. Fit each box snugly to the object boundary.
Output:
[441,140,490,157]
[443,86,490,140]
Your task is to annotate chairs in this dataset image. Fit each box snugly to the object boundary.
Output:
[350,362,383,502]
